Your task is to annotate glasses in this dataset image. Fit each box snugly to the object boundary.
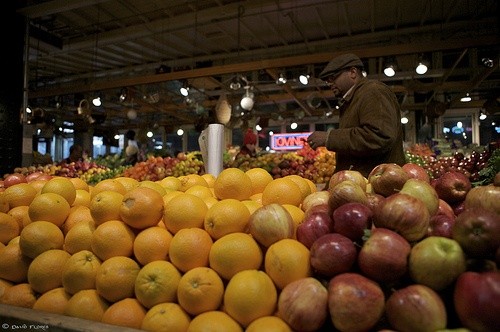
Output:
[324,70,345,83]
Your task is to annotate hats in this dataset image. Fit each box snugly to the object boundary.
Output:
[319,53,363,81]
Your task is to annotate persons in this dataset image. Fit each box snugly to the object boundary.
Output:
[65,145,82,161]
[308,54,405,168]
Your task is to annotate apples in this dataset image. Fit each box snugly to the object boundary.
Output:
[371,191,431,239]
[306,203,331,224]
[357,227,411,284]
[277,278,328,331]
[453,270,500,332]
[408,235,466,291]
[434,199,454,220]
[433,172,471,205]
[452,203,465,217]
[326,272,385,332]
[328,180,368,211]
[247,204,293,247]
[452,209,500,253]
[310,234,358,279]
[399,178,439,216]
[368,162,404,196]
[328,166,366,196]
[364,182,375,196]
[302,190,328,214]
[332,201,372,235]
[296,211,333,249]
[427,214,453,237]
[402,163,430,187]
[465,184,500,225]
[384,284,448,331]
